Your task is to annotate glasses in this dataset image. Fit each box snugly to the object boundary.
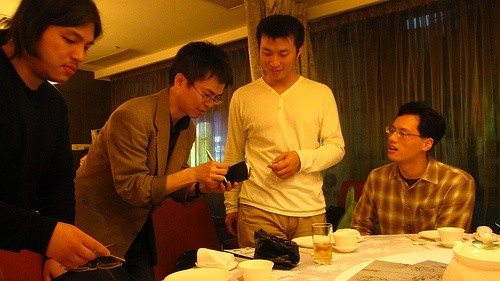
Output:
[385,125,421,140]
[60,243,126,271]
[192,84,224,106]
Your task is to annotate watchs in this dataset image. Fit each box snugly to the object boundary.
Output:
[195,181,204,197]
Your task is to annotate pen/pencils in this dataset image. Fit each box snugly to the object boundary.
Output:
[206,150,228,183]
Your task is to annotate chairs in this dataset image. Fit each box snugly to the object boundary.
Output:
[337,179,365,207]
[151,194,220,281]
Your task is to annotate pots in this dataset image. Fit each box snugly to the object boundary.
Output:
[442,236,500,281]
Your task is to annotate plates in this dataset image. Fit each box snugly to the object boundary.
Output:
[419,230,440,240]
[290,236,313,248]
[332,243,360,252]
[437,240,464,248]
[163,268,232,281]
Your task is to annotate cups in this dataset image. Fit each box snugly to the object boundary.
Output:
[333,229,363,247]
[238,259,274,281]
[438,227,465,242]
[311,223,333,264]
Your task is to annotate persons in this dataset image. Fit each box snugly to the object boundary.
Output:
[348,101,477,237]
[76,41,241,281]
[223,14,345,249]
[0,0,114,281]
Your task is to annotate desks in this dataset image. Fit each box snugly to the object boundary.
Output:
[162,234,500,281]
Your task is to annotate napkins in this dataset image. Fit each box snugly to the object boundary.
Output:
[195,247,238,271]
